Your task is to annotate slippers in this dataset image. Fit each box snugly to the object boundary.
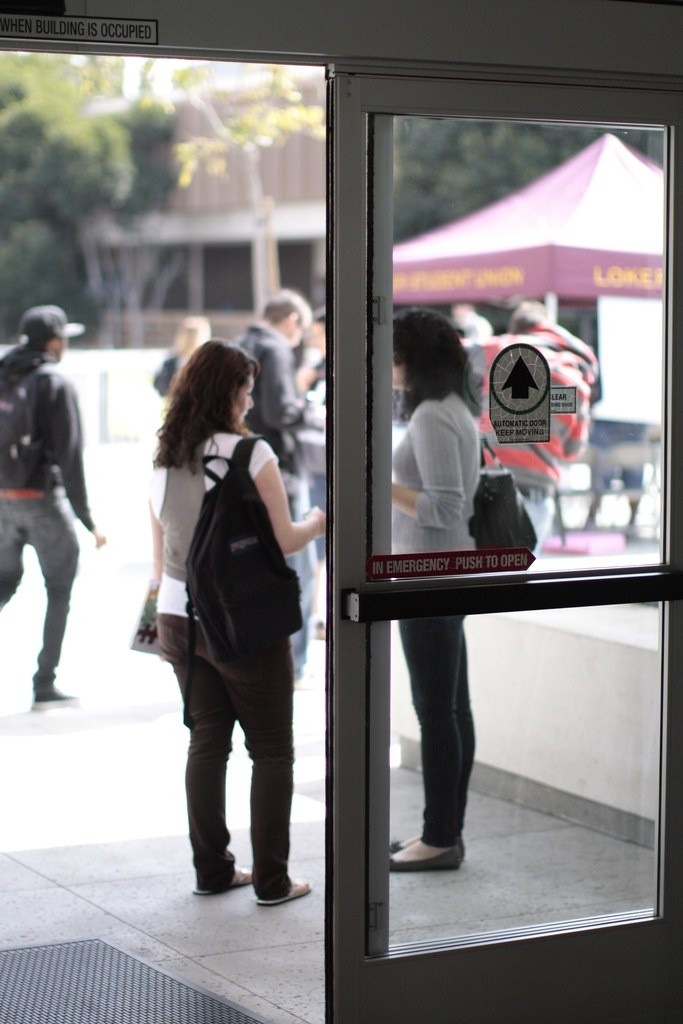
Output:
[192,867,251,895]
[257,880,314,906]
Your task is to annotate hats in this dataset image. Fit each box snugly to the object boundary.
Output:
[19,304,85,339]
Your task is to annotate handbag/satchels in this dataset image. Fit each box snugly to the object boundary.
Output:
[467,437,538,554]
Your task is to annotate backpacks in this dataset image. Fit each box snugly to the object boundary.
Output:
[182,434,303,731]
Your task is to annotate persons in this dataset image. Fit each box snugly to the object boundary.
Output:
[151,288,645,688]
[144,339,327,907]
[0,302,110,710]
[388,314,485,868]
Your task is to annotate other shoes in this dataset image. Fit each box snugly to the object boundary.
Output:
[33,689,80,709]
[390,836,466,871]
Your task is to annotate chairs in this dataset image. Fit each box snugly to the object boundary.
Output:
[553,425,665,548]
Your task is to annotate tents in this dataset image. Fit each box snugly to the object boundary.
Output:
[390,130,664,325]
